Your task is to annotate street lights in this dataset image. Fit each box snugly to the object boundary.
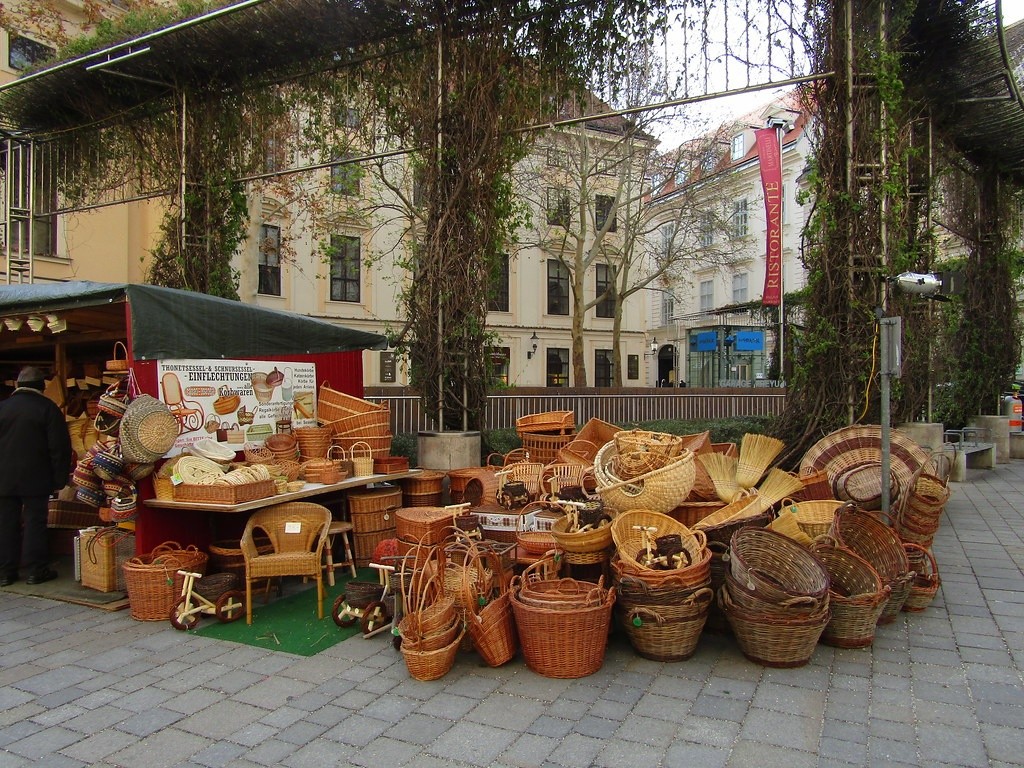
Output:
[766,116,795,386]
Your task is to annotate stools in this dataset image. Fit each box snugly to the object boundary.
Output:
[510,546,548,581]
[305,519,357,587]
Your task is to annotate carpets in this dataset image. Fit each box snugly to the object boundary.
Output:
[0,553,132,604]
[191,569,387,657]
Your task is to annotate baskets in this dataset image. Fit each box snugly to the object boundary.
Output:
[60,345,954,674]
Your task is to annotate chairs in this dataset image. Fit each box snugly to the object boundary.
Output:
[161,372,204,437]
[470,461,583,542]
[276,410,291,434]
[240,501,333,624]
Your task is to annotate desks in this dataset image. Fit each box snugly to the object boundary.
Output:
[143,469,421,596]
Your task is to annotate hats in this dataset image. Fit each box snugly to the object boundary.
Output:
[16,365,46,382]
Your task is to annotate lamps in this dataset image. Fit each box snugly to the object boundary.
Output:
[527,330,539,359]
[3,311,69,334]
[644,336,658,360]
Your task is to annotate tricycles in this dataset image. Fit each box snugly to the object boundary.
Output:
[168,570,247,631]
[331,563,396,634]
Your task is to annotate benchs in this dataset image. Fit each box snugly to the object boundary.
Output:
[943,428,996,482]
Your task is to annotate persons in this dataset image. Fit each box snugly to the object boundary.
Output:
[662,379,673,387]
[680,380,686,388]
[0,367,72,587]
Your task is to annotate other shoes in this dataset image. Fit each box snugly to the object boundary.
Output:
[26,568,58,585]
[1,572,20,586]
